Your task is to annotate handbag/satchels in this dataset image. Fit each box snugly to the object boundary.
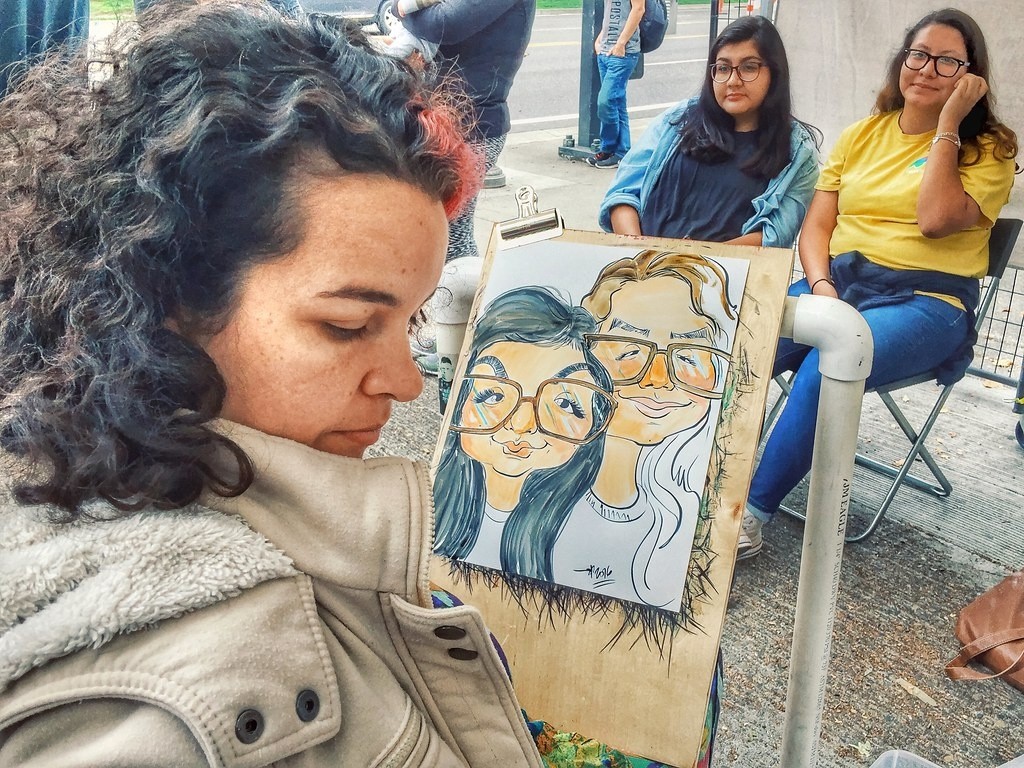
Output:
[945,568,1024,693]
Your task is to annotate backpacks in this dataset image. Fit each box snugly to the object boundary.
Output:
[630,0,668,53]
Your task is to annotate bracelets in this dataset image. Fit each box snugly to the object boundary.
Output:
[932,132,961,150]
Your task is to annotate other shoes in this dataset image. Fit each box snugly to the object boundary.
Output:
[411,336,436,356]
[414,357,439,375]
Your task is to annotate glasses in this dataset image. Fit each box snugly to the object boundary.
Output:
[708,61,768,83]
[904,47,971,78]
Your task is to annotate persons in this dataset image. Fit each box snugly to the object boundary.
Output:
[730,8,1019,568]
[391,0,536,372]
[367,0,445,67]
[599,14,821,251]
[589,0,669,169]
[0,0,541,768]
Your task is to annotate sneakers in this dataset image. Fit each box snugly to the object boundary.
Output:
[595,154,621,169]
[735,508,764,561]
[586,153,614,166]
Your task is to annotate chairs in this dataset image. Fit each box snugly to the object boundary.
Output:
[755,219,1024,543]
